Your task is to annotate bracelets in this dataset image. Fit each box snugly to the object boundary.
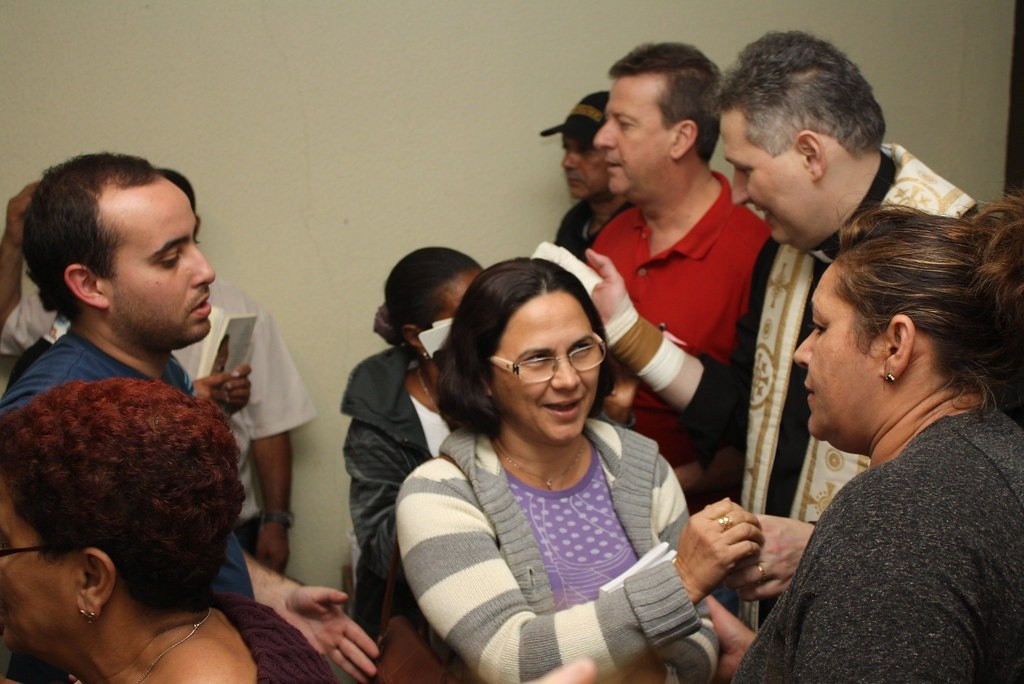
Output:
[261,512,294,529]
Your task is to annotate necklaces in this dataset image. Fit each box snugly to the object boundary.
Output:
[417,368,440,414]
[496,436,585,491]
[138,608,211,684]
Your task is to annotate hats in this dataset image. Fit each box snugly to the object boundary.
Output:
[539,91,609,141]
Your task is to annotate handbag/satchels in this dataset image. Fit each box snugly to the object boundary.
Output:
[369,457,500,684]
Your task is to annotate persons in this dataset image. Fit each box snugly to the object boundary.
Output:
[0,168,315,574]
[393,256,765,684]
[0,379,338,684]
[540,90,634,261]
[703,193,1024,684]
[533,30,978,630]
[586,42,771,510]
[0,312,251,423]
[339,246,487,684]
[0,155,379,684]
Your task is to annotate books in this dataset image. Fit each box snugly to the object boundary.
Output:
[197,314,258,379]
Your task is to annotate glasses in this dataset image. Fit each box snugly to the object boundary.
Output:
[487,332,606,383]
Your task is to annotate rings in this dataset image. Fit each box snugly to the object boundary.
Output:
[758,565,766,581]
[718,515,733,530]
